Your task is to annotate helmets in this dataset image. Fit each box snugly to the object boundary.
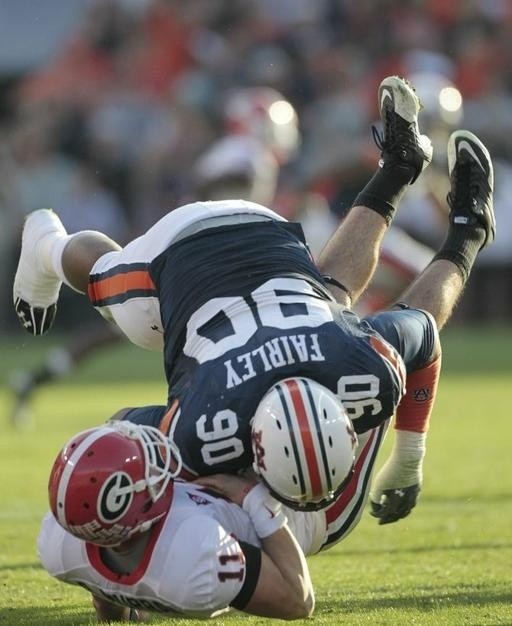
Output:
[249,376,357,511]
[48,421,174,548]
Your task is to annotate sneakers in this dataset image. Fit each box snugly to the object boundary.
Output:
[377,75,433,185]
[447,129,496,252]
[12,209,65,336]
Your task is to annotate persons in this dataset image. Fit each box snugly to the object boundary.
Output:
[1,2,511,336]
[35,74,496,620]
[13,200,443,622]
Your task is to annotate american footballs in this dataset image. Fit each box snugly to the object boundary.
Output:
[92,595,149,622]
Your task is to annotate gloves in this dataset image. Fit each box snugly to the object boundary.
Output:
[369,430,426,525]
